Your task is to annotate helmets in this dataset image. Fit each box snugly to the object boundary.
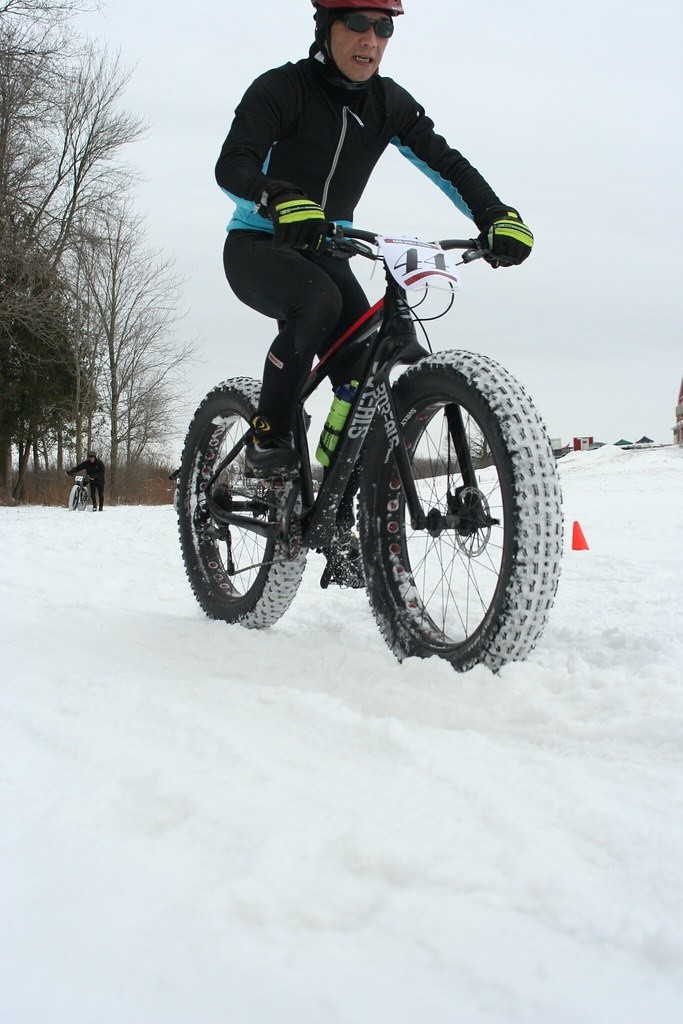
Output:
[87,451,97,457]
[310,0,405,17]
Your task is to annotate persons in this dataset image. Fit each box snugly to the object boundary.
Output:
[66,451,106,511]
[215,0,535,589]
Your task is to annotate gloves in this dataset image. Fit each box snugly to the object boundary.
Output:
[479,205,533,269]
[254,181,328,256]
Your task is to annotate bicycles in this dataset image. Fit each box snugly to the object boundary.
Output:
[68,473,90,511]
[176,203,563,674]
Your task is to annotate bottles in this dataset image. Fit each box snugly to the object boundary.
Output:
[316,379,359,465]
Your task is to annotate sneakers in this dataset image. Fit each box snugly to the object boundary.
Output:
[242,412,302,481]
[322,528,366,589]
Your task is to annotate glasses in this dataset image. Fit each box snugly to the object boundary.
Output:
[335,13,394,38]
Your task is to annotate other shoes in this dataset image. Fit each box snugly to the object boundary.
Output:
[92,503,97,511]
[99,506,102,511]
